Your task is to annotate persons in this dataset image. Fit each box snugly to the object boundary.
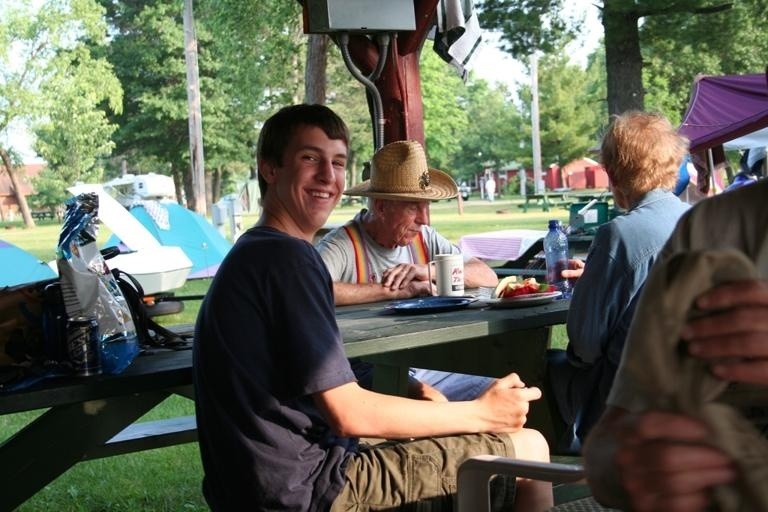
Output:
[547,108,768,512]
[192,102,556,512]
[485,176,496,202]
[315,139,502,402]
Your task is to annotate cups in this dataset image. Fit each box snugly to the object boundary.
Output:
[428,254,464,297]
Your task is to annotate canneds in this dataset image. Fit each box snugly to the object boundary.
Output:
[66,317,102,376]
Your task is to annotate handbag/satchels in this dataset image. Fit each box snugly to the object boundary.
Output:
[0,269,147,389]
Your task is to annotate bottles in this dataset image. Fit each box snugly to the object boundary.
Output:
[544,220,570,299]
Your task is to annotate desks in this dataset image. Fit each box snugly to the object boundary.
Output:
[518,192,614,213]
[460,228,547,268]
[0,287,574,512]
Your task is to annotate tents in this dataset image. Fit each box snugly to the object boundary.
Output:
[96,199,235,279]
[0,240,56,287]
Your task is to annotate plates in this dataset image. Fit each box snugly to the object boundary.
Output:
[484,291,561,309]
[385,297,478,314]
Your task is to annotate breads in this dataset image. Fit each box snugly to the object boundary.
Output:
[492,275,516,298]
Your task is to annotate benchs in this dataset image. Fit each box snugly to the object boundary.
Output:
[83,412,199,460]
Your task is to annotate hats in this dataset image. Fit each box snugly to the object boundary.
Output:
[342,139,459,201]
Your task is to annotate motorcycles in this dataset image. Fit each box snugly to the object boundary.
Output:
[459,183,470,201]
